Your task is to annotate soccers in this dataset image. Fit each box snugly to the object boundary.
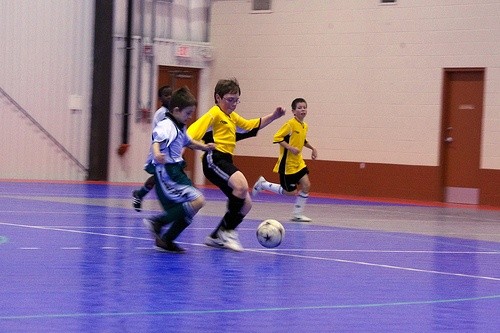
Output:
[256,218,285,248]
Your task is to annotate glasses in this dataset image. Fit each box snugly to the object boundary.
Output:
[222,96,240,104]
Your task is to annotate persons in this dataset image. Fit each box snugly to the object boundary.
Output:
[187,80,286,253]
[143,87,214,253]
[133,85,174,211]
[252,98,317,222]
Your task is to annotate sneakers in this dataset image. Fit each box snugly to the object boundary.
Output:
[252,177,265,197]
[217,230,241,251]
[144,217,163,239]
[156,240,186,253]
[204,236,226,248]
[132,190,142,212]
[290,214,311,222]
